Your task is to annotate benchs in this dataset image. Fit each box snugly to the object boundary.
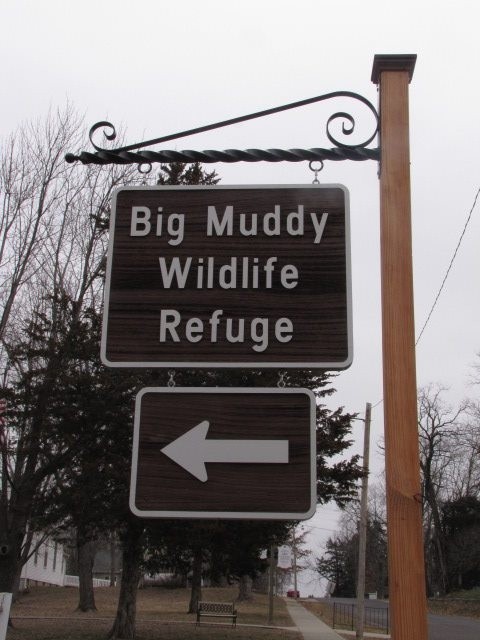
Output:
[196,600,238,629]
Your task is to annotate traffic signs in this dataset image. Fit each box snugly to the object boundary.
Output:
[102,178,360,522]
